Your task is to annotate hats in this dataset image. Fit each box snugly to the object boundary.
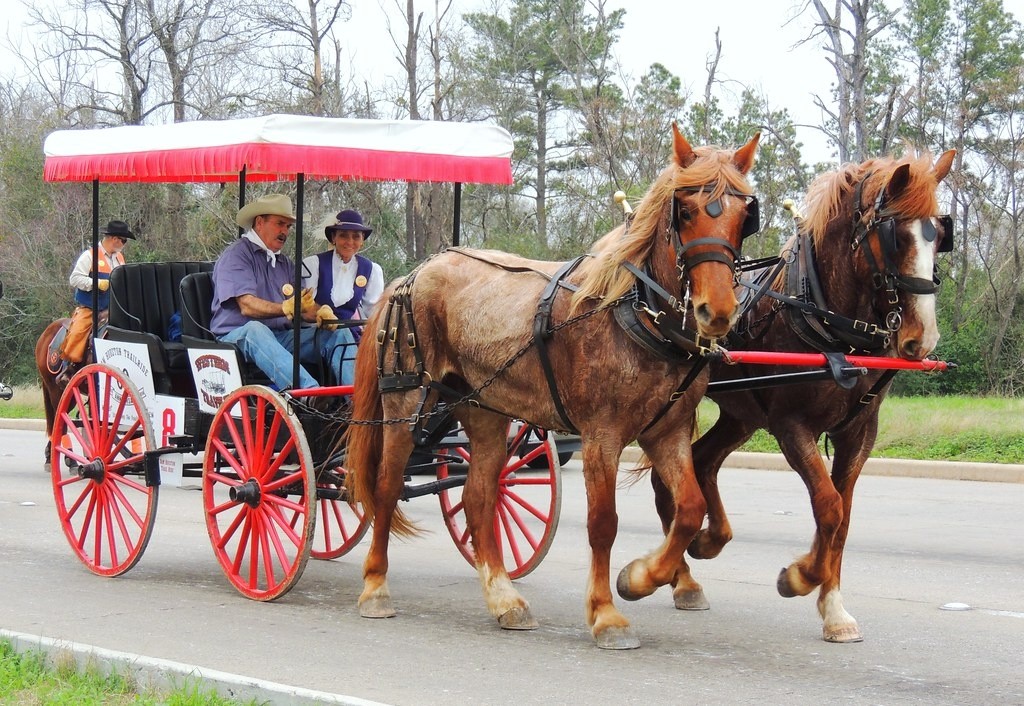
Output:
[100,220,136,240]
[325,210,373,245]
[235,194,311,228]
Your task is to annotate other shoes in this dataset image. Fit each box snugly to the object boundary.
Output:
[59,366,75,386]
[336,397,348,410]
[306,387,334,412]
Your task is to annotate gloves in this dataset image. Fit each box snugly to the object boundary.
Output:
[316,305,339,331]
[98,279,109,291]
[282,287,315,320]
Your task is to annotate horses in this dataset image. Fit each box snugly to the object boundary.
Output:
[348,121,956,650]
[33,315,110,474]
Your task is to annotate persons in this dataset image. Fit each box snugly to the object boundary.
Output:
[302,210,383,346]
[57,220,137,382]
[209,192,358,405]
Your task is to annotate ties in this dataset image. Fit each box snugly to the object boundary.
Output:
[105,253,114,268]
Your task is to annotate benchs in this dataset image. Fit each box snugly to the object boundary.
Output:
[178,272,330,408]
[108,261,216,396]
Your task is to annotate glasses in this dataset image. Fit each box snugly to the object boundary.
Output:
[112,236,127,245]
